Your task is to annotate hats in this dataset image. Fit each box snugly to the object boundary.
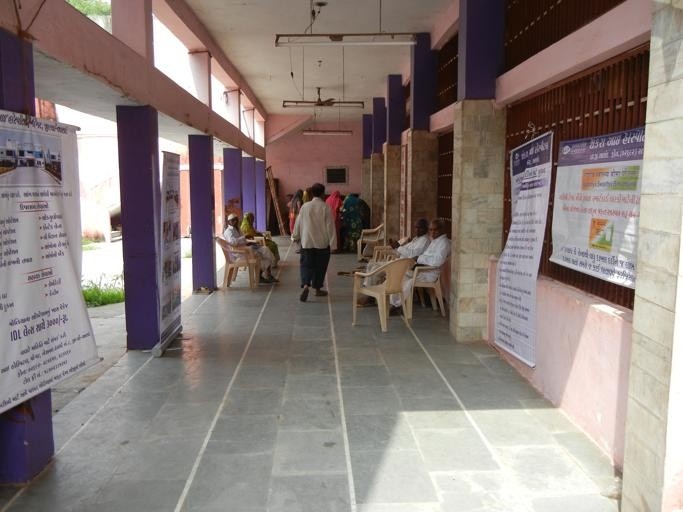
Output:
[227,213,238,221]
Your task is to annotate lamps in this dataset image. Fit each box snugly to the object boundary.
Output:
[274,0,418,45]
[281,44,364,108]
[303,106,352,135]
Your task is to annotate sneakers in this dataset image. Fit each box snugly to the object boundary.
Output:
[354,296,376,307]
[389,306,402,316]
[300,287,328,301]
[258,275,280,286]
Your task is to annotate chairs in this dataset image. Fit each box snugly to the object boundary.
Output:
[352,225,446,333]
[215,231,271,288]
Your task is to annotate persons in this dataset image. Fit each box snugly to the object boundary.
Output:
[287,189,304,235]
[357,217,451,314]
[325,190,370,252]
[291,183,338,302]
[224,212,280,285]
